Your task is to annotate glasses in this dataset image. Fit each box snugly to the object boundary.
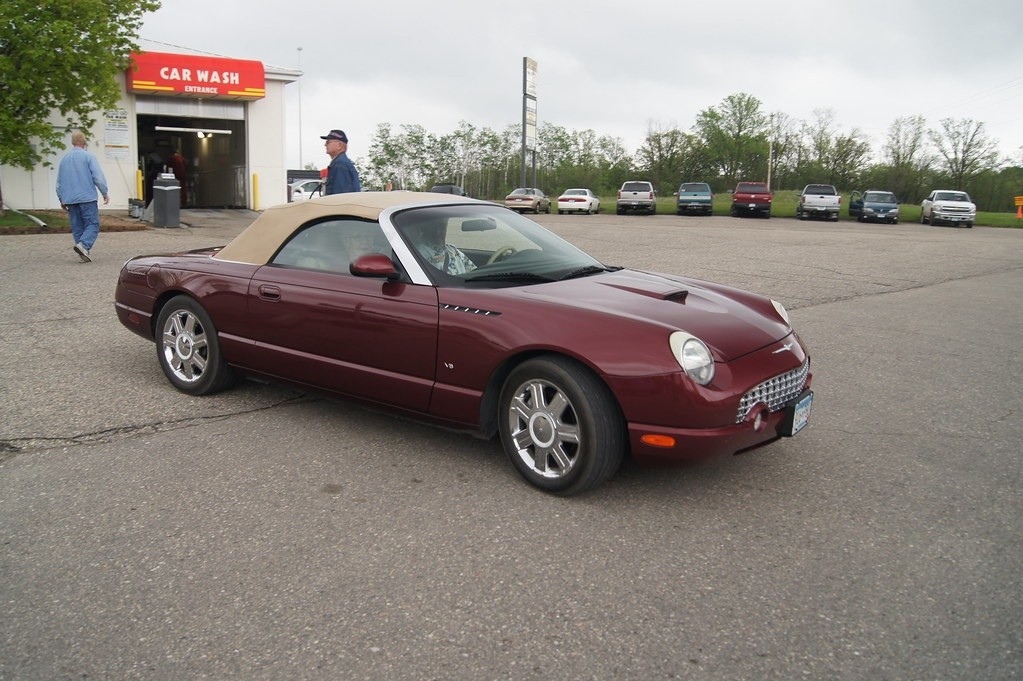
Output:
[326,141,340,144]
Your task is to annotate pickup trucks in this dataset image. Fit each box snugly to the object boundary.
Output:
[673,182,715,216]
[616,180,656,214]
[797,184,841,221]
[727,182,771,218]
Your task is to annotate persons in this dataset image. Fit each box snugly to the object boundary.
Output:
[417,220,477,275]
[149,150,188,208]
[56,133,109,262]
[320,130,361,195]
[857,197,862,221]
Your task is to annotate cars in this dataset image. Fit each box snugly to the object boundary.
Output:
[288,178,325,201]
[557,189,601,215]
[114,189,813,498]
[505,188,552,214]
[851,189,900,223]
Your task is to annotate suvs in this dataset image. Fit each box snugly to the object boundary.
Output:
[921,189,977,228]
[431,184,469,198]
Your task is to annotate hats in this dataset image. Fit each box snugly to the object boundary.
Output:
[320,130,347,144]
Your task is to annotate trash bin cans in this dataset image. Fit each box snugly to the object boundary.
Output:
[152,179,182,229]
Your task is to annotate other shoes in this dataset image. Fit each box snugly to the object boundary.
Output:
[73,243,92,262]
